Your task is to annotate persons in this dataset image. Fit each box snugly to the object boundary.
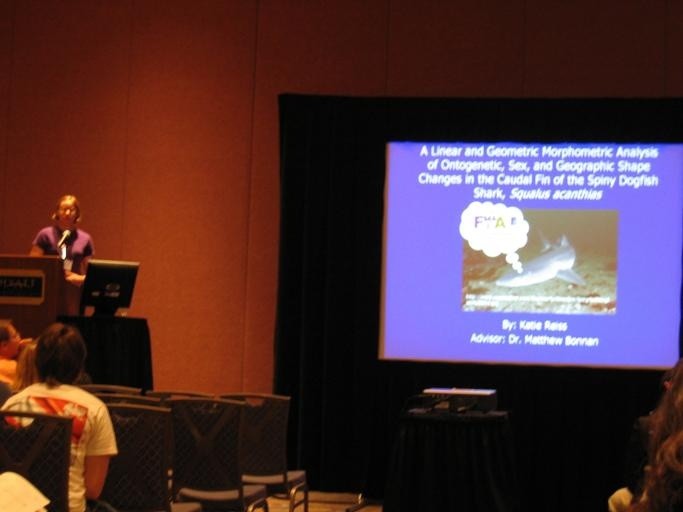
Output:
[0,318,119,512]
[607,358,683,511]
[29,195,96,316]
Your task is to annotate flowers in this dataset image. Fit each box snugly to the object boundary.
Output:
[58,229,71,248]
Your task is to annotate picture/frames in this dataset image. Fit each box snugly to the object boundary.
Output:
[57,315,154,396]
[383,408,522,512]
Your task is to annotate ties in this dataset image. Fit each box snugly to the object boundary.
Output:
[80,259,139,320]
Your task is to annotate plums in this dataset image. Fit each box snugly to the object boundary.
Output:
[424,387,496,412]
